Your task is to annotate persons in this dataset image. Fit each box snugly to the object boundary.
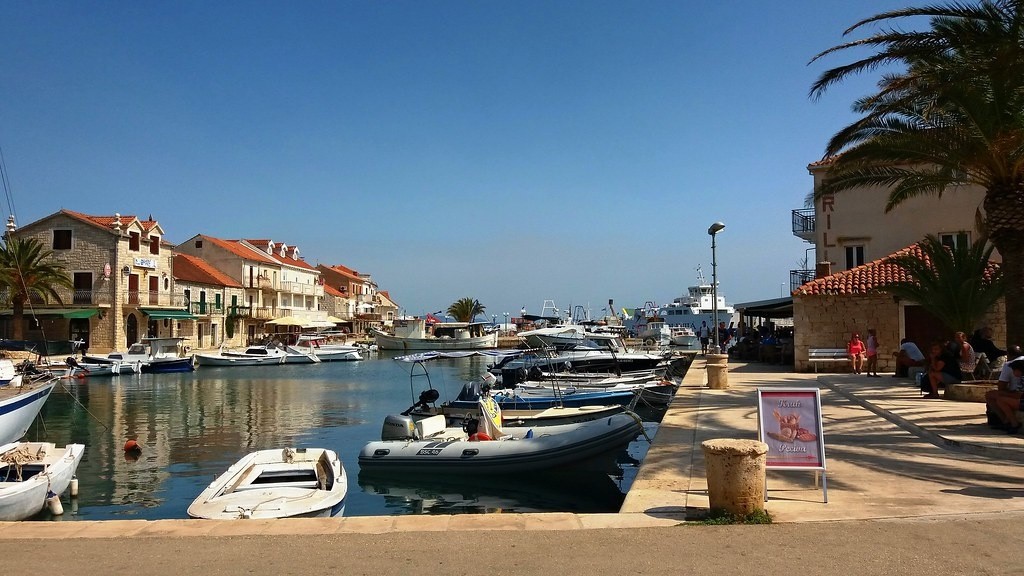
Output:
[846,332,865,374]
[866,330,880,378]
[696,320,711,357]
[892,339,923,377]
[925,328,1007,399]
[996,345,1024,430]
[713,318,794,357]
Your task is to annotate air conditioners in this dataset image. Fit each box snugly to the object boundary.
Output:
[340,286,347,291]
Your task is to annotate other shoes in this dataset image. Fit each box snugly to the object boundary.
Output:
[892,375,901,377]
[701,354,704,356]
[1001,422,1022,434]
[858,369,862,374]
[853,369,856,374]
[867,374,881,377]
[991,423,1005,429]
[924,392,940,398]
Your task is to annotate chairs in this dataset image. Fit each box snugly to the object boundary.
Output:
[759,345,778,363]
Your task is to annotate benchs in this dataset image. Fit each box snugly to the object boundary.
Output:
[235,480,319,492]
[808,348,868,373]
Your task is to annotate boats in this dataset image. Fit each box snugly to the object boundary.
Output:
[636,302,671,346]
[623,264,756,338]
[290,331,364,361]
[448,326,689,415]
[0,349,61,446]
[669,324,696,346]
[358,351,643,476]
[187,446,348,521]
[0,440,85,522]
[105,337,194,372]
[33,355,143,378]
[369,308,499,350]
[195,346,321,365]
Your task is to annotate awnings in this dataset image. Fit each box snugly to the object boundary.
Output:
[138,307,199,320]
[0,303,112,324]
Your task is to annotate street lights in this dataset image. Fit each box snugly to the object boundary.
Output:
[503,312,509,332]
[492,315,498,326]
[708,220,726,354]
[445,316,450,323]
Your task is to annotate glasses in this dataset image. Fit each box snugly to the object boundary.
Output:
[854,337,859,338]
